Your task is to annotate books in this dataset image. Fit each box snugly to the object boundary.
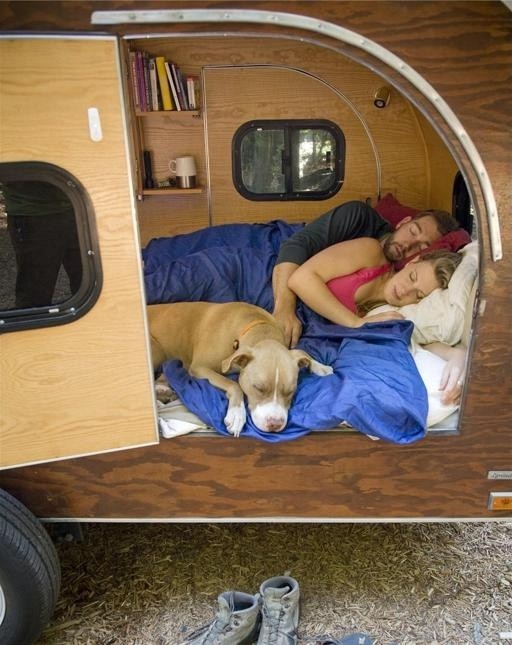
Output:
[128,49,201,113]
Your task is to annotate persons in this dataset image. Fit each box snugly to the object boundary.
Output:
[285,235,469,407]
[270,199,462,349]
[0,178,84,309]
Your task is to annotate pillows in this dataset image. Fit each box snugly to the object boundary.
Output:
[366,240,481,346]
[373,193,468,270]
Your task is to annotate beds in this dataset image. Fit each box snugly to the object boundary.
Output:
[139,173,482,434]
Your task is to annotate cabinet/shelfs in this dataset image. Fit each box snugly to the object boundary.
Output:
[118,39,205,201]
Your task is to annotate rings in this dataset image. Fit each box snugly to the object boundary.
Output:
[458,379,463,389]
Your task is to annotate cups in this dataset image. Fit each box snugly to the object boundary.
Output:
[168,156,198,189]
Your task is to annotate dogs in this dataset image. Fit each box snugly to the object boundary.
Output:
[145,300,335,438]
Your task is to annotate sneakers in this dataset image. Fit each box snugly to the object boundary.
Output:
[189,590,261,645]
[256,576,302,645]
